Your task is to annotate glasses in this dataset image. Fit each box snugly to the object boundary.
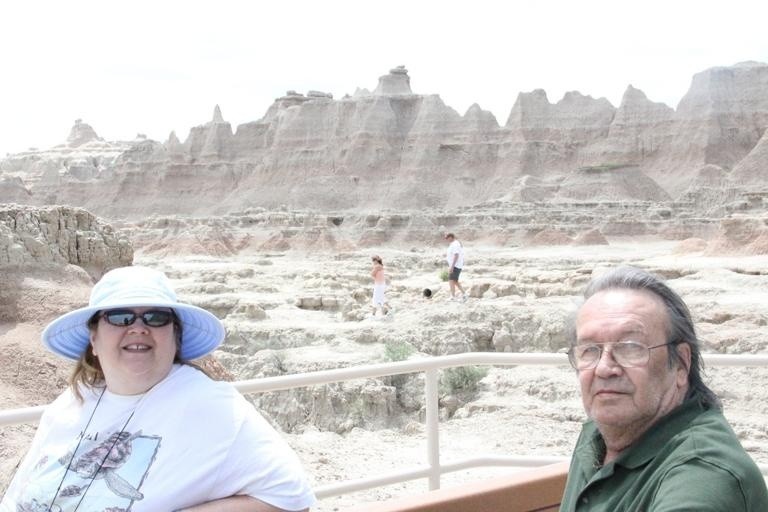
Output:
[565,341,675,371]
[97,309,175,327]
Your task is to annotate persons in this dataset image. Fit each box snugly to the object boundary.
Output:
[0,265,317,512]
[443,232,468,301]
[559,265,767,511]
[370,255,387,315]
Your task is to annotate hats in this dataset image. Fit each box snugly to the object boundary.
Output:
[42,266,225,361]
